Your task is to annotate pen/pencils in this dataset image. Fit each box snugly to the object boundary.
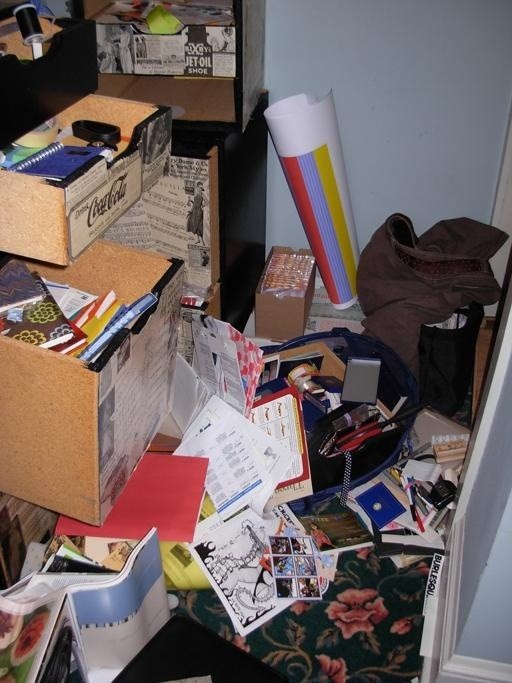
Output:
[390,442,432,532]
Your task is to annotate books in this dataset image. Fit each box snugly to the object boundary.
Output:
[1,138,314,683]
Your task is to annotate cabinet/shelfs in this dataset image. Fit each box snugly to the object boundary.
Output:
[73,1,266,133]
[170,89,269,332]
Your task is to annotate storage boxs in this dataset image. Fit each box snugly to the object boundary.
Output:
[255,246,316,341]
